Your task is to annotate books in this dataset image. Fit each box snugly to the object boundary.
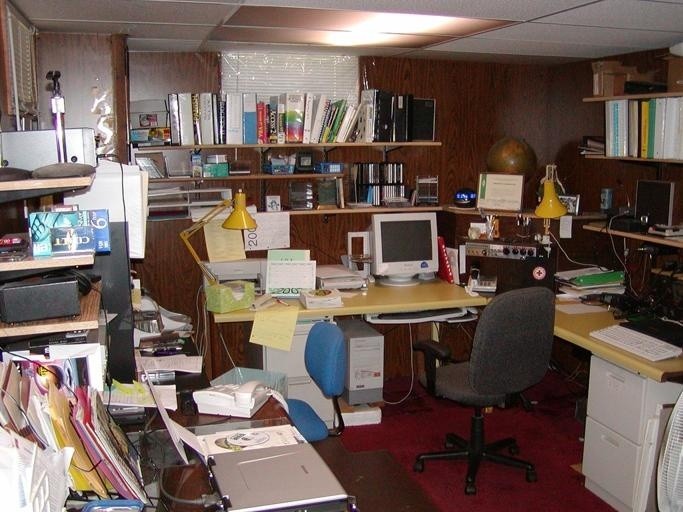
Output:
[167,91,374,145]
[438,237,453,283]
[25,376,147,504]
[605,97,683,161]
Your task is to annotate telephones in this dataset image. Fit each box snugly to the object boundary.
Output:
[467,269,497,292]
[192,380,270,418]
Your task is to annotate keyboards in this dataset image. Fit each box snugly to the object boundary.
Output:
[588,324,683,362]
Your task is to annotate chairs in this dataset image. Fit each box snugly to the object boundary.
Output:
[281,321,348,443]
[414,287,557,496]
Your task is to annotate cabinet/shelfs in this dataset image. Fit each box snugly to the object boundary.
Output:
[581,91,683,283]
[0,168,104,341]
[131,141,445,222]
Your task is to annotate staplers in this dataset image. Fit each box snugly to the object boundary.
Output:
[252,294,276,311]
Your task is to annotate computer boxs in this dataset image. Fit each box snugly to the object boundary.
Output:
[337,320,385,407]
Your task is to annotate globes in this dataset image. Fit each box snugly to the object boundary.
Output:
[486,136,536,186]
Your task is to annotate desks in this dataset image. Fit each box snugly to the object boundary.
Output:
[553,284,683,512]
[204,274,489,429]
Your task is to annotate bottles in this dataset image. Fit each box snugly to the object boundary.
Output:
[469,261,481,287]
[601,187,615,210]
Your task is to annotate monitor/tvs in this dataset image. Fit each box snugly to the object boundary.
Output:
[634,179,675,229]
[367,211,439,287]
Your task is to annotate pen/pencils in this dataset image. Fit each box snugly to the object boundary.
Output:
[516,214,532,227]
[278,299,290,306]
[486,215,495,240]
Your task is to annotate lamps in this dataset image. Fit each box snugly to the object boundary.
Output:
[179,189,258,286]
[534,164,570,235]
